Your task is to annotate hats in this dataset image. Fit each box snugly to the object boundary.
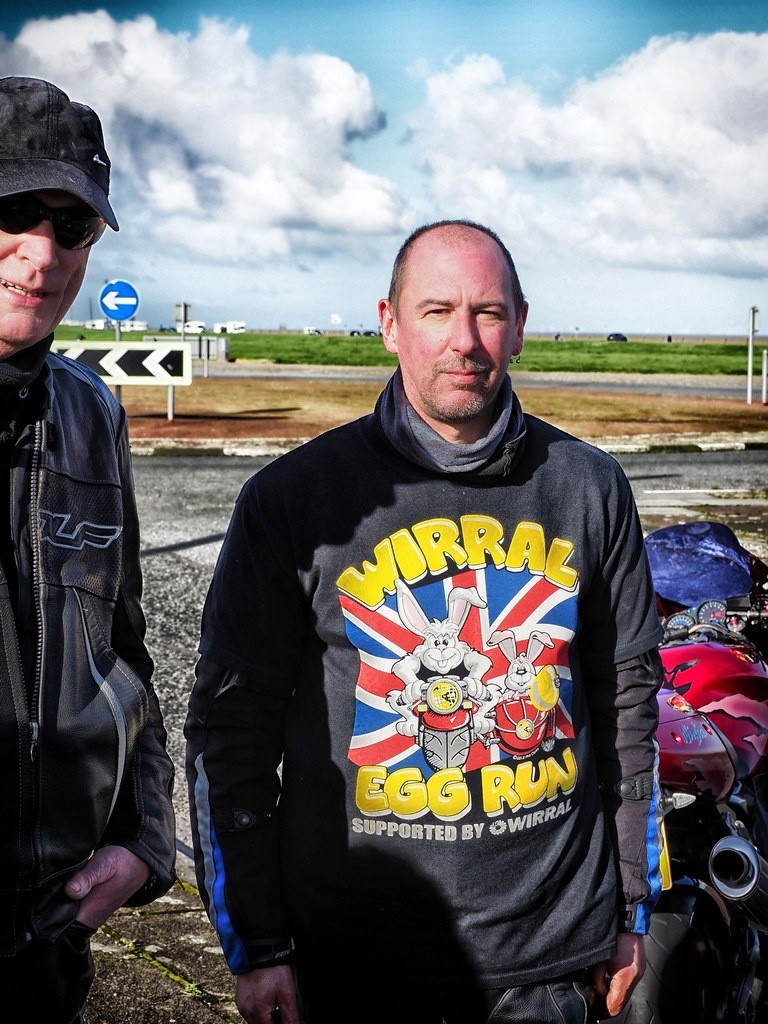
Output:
[0,77,119,232]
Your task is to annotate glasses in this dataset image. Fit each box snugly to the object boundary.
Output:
[0,192,106,250]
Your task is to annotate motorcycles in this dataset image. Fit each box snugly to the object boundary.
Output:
[604,522,768,1021]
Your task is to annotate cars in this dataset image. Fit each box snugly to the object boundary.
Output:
[607,334,627,343]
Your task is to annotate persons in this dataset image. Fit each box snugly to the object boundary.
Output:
[0,77,184,1024]
[182,218,666,1024]
